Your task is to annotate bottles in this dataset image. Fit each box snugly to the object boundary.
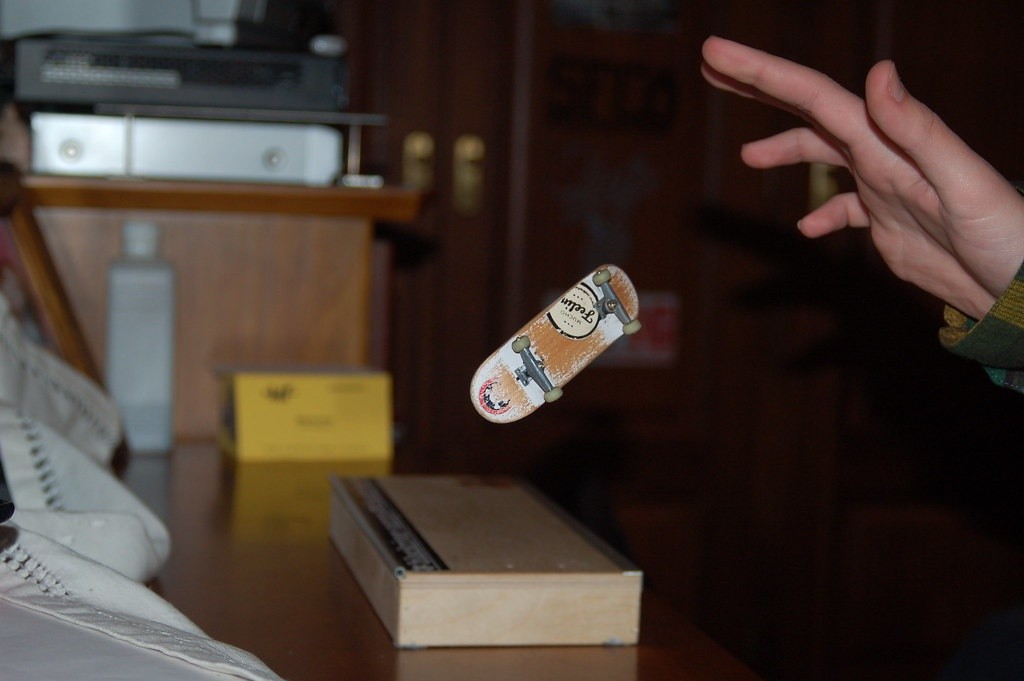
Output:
[102,219,175,454]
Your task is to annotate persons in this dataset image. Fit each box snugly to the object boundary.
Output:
[702,32,1024,394]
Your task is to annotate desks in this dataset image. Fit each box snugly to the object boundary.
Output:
[110,437,755,681]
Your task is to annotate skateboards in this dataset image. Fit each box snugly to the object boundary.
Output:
[470,263,642,425]
